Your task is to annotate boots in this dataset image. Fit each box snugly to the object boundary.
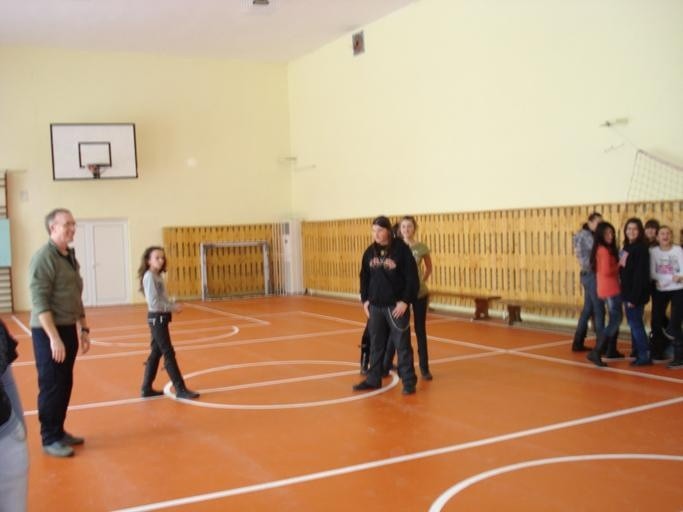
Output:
[587,335,609,366]
[143,358,163,396]
[361,344,370,375]
[164,359,200,398]
[607,336,624,358]
[572,331,592,352]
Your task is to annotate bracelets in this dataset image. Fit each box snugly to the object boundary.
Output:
[80,328,89,334]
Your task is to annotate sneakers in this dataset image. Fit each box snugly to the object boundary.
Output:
[655,355,663,360]
[631,358,652,366]
[667,359,683,368]
[403,385,416,394]
[43,442,74,456]
[352,381,381,390]
[60,434,84,444]
[420,367,432,379]
[382,369,389,376]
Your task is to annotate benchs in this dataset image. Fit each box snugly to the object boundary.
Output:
[505,297,603,327]
[428,289,501,320]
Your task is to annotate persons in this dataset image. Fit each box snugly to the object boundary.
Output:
[586,221,625,366]
[616,217,653,365]
[571,211,605,352]
[26,208,90,458]
[397,216,432,379]
[352,216,419,395]
[643,219,660,247]
[0,317,31,511]
[647,225,683,368]
[360,223,403,375]
[136,247,200,398]
